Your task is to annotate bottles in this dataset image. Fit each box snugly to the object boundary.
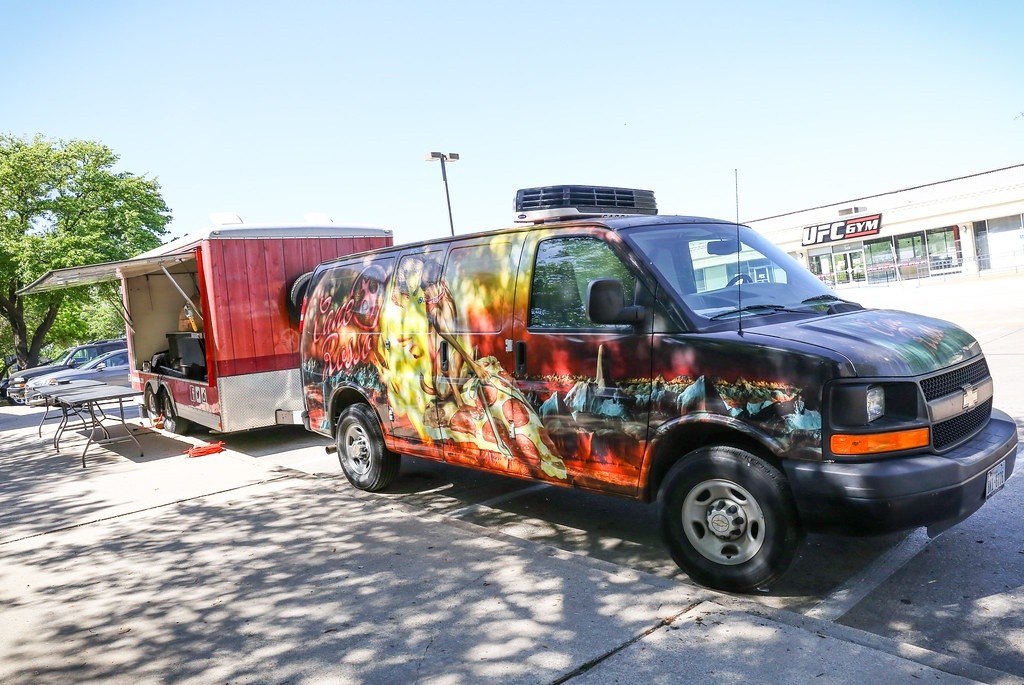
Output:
[142,360,152,373]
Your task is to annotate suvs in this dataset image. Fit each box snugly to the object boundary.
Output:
[298,183,1021,595]
[6,337,129,407]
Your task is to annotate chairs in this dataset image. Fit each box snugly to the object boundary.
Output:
[533,252,586,326]
[636,246,683,310]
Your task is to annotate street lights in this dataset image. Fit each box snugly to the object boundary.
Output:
[428,150,461,239]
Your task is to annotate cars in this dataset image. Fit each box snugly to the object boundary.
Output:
[25,348,130,409]
[0,357,86,409]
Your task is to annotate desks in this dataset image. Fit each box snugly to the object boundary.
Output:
[33,379,144,469]
